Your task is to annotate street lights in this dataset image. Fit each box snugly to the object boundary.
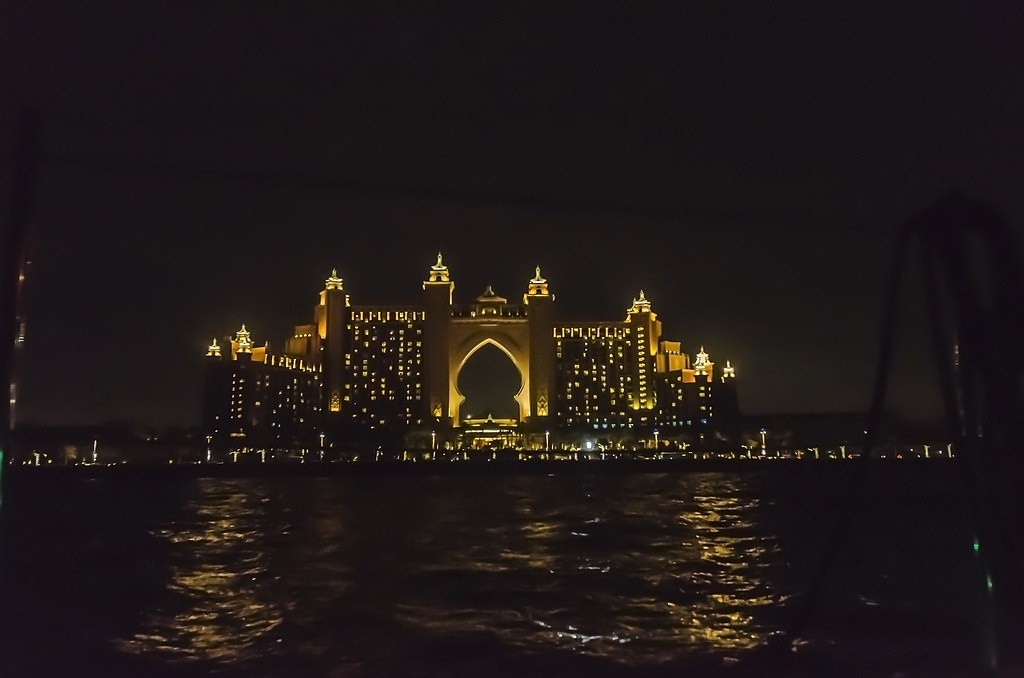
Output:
[203,434,213,461]
[541,430,552,459]
[92,438,100,464]
[427,428,439,460]
[757,428,770,458]
[317,430,328,460]
[652,429,662,455]
